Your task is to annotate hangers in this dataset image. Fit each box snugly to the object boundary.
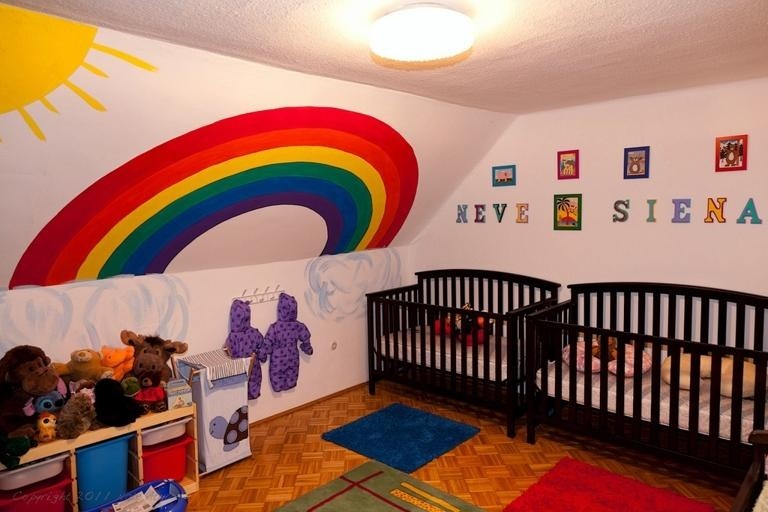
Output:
[233,284,285,306]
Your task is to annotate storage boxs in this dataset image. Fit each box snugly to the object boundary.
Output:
[174,349,257,476]
[75,432,134,512]
[1,474,70,512]
[142,437,193,489]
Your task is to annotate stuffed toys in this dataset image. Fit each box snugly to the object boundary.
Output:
[38,411,57,443]
[52,345,167,440]
[120,329,189,387]
[0,344,59,440]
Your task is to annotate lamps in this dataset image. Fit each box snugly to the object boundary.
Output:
[362,0,483,70]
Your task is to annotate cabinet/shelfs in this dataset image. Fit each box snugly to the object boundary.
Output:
[0,402,200,511]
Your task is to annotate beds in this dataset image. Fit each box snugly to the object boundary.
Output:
[525,281,768,484]
[364,268,560,438]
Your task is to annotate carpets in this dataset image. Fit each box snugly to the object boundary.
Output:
[502,455,721,512]
[271,459,490,511]
[322,401,482,474]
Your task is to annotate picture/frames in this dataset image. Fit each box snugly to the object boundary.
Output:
[715,135,747,171]
[624,146,649,179]
[557,149,579,180]
[553,193,582,230]
[492,165,516,186]
